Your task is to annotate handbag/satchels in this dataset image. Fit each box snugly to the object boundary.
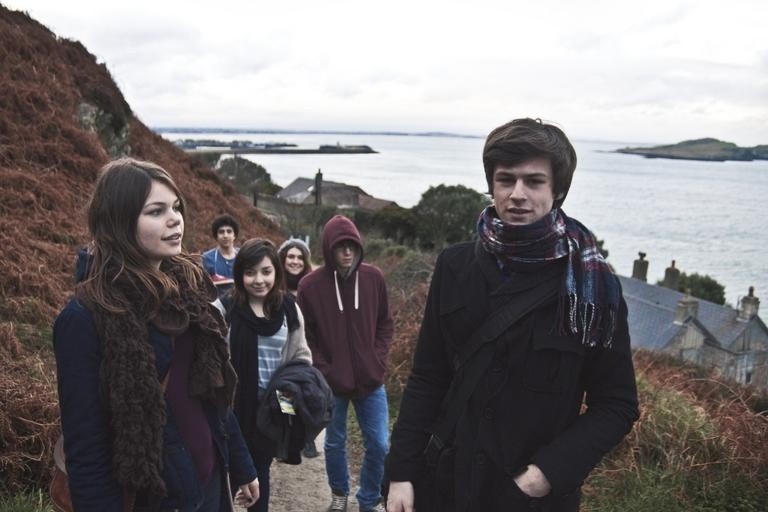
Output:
[49,432,138,512]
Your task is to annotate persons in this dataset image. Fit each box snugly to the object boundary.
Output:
[54,157,395,511]
[381,121,640,510]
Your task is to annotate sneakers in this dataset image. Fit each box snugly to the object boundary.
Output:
[326,492,348,512]
[358,505,386,512]
